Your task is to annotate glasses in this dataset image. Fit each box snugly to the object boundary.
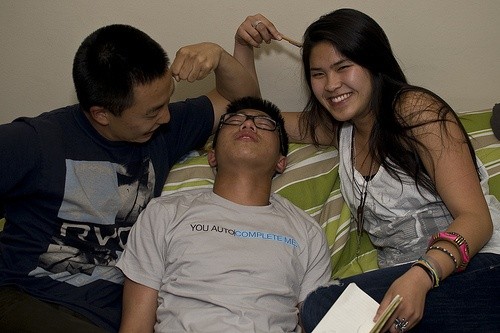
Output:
[219,112,281,131]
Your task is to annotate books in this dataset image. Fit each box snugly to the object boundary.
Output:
[310,283,405,333]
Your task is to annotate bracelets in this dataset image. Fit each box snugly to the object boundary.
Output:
[427,231,470,271]
[411,254,443,287]
[425,245,458,272]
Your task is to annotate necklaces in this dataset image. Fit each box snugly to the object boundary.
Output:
[351,127,374,244]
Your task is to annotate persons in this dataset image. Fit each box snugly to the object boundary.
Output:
[118,96,332,332]
[233,6,500,333]
[1,22,263,333]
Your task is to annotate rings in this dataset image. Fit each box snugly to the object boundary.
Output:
[252,19,262,28]
[394,318,409,333]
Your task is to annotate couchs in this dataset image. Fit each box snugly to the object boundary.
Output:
[160,105,500,282]
